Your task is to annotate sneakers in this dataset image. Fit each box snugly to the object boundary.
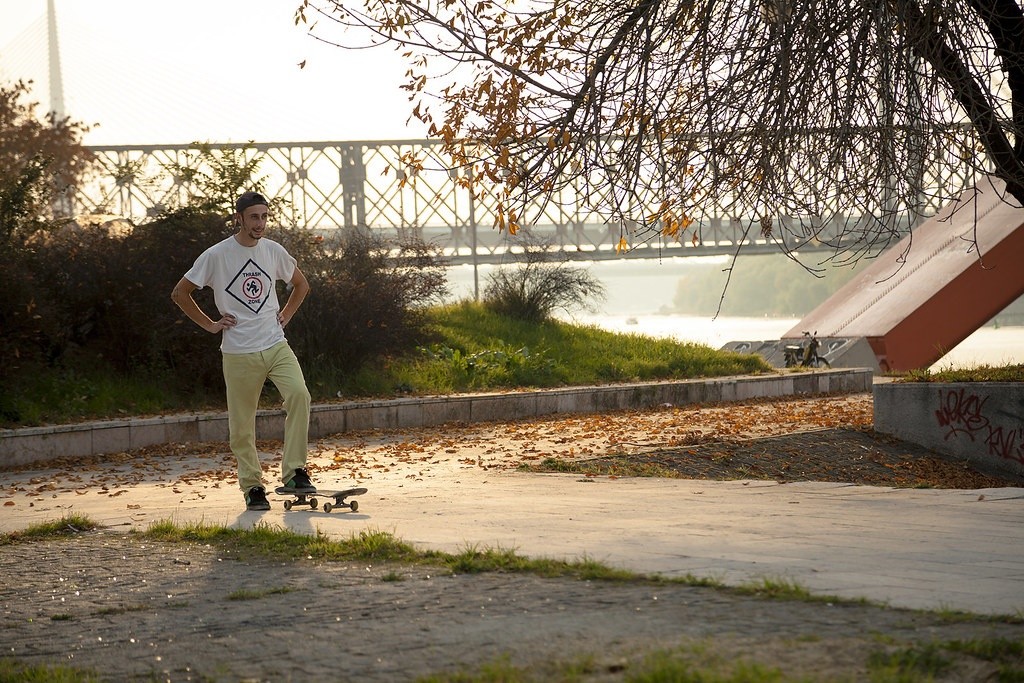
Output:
[283,468,317,493]
[245,485,270,510]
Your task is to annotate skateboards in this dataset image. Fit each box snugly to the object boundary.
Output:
[273,484,368,512]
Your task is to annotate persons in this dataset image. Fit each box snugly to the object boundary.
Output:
[170,192,316,512]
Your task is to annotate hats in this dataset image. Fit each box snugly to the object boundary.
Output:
[235,191,271,227]
